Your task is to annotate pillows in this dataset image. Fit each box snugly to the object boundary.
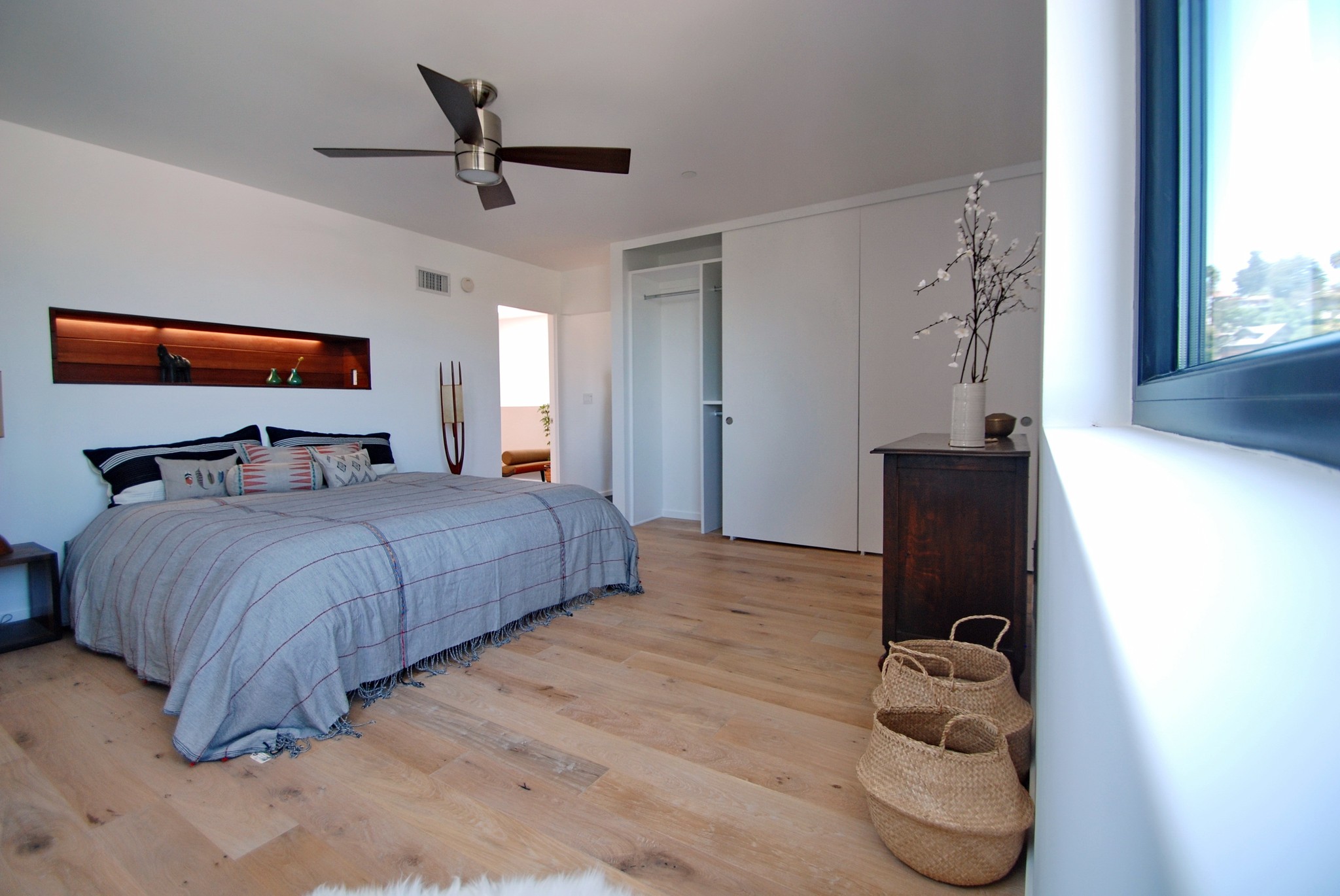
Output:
[82,424,396,510]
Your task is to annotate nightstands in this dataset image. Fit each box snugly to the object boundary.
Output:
[0,541,63,655]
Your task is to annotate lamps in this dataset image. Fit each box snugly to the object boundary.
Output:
[440,361,465,475]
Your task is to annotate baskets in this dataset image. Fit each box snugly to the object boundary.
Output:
[855,654,1036,886]
[872,614,1034,793]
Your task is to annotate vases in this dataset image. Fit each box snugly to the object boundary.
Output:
[287,369,303,385]
[949,382,986,447]
[267,368,282,384]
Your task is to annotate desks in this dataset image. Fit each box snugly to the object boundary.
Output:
[870,432,1031,679]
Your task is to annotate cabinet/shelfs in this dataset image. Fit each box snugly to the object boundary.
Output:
[610,159,1043,555]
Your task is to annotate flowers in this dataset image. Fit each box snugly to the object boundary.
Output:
[912,172,1041,384]
[295,356,304,368]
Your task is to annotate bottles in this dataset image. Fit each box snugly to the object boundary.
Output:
[266,368,282,385]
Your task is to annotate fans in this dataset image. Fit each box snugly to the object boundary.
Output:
[313,64,631,211]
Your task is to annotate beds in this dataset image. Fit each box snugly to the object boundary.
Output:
[55,473,642,765]
